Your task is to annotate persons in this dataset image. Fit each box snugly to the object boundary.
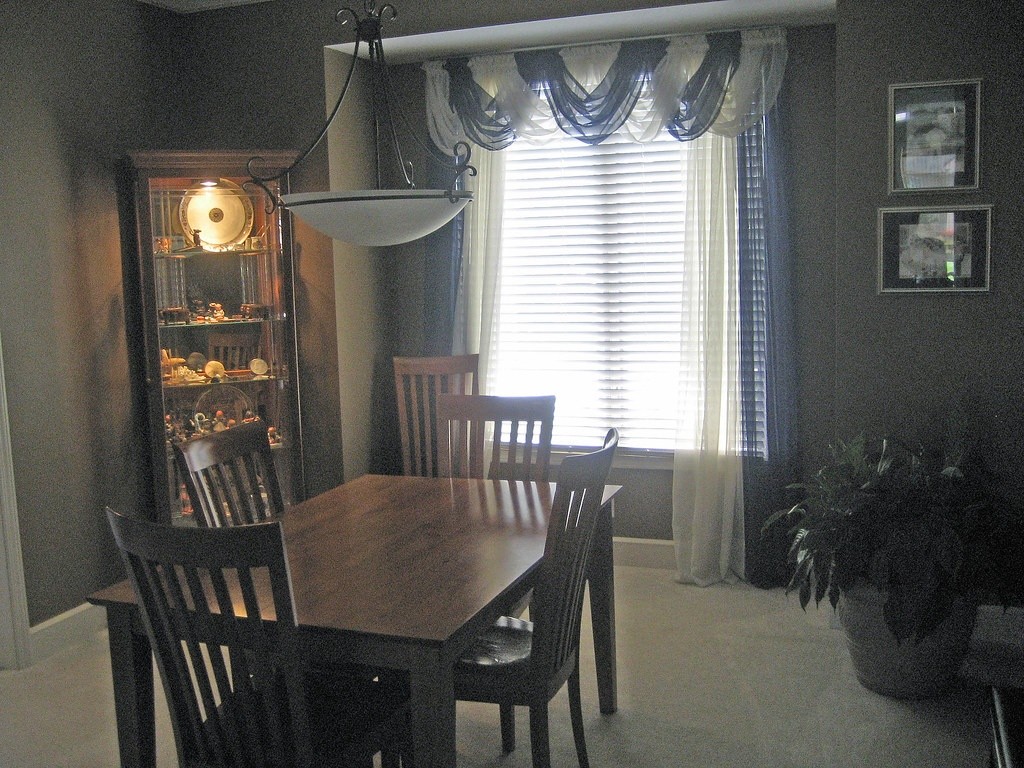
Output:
[923,123,948,173]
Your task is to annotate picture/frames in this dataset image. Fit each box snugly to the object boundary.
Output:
[877,204,992,298]
[886,76,984,196]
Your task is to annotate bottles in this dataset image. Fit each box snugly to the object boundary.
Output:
[196,301,205,323]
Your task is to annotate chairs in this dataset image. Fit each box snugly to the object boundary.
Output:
[435,393,554,483]
[377,429,618,768]
[105,506,409,768]
[393,353,482,477]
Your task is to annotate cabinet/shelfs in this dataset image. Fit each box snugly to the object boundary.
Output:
[112,146,307,560]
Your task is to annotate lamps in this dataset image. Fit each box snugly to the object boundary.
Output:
[241,0,478,246]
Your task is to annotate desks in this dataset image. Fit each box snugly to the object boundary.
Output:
[86,474,624,768]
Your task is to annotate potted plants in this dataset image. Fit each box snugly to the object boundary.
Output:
[764,434,1024,699]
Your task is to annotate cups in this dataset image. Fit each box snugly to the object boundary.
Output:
[251,236,264,251]
[245,238,252,251]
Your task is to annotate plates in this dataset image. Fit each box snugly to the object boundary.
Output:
[250,358,268,374]
[179,178,254,252]
